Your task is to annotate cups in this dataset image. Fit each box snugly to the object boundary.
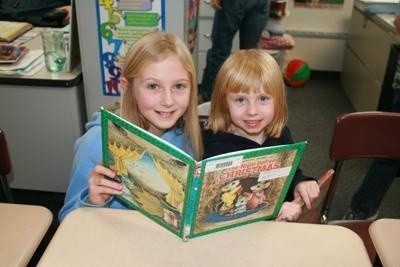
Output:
[40,31,67,72]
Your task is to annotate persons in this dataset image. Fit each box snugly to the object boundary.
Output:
[201,46,320,222]
[57,29,206,226]
[199,0,289,102]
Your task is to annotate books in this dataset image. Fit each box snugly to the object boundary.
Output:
[100,105,308,243]
[0,19,46,78]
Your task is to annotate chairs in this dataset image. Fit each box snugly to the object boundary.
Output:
[315,110,400,264]
[0,129,12,202]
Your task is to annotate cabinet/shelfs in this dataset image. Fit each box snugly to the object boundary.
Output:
[0,64,85,194]
[338,7,400,111]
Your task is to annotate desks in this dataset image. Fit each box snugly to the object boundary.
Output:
[1,203,53,267]
[368,218,400,264]
[37,206,373,266]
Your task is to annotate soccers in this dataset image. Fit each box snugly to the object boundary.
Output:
[284,59,310,88]
